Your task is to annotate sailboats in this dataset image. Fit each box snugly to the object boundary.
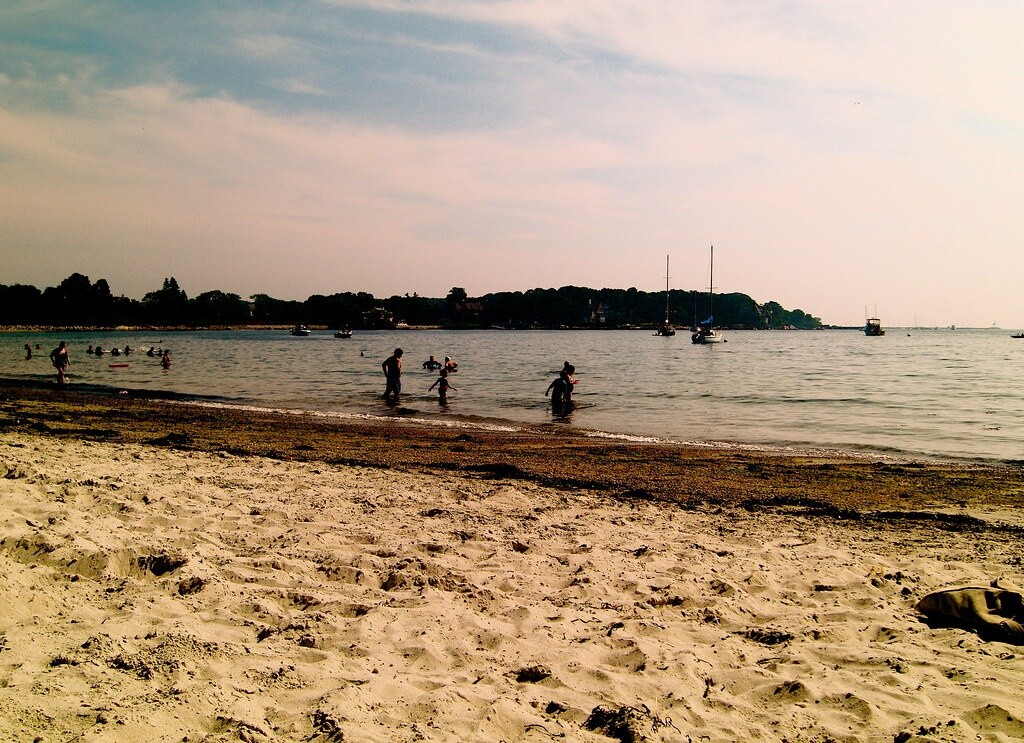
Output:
[690,291,700,332]
[699,245,723,343]
[657,255,676,336]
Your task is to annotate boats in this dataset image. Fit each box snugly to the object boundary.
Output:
[333,329,353,338]
[864,318,886,336]
[1011,333,1024,338]
[288,327,312,336]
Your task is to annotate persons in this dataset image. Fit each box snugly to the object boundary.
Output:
[35,344,40,349]
[24,344,32,359]
[381,348,403,399]
[423,356,442,370]
[50,341,71,382]
[428,369,457,396]
[445,356,458,370]
[545,361,580,404]
[87,345,172,366]
